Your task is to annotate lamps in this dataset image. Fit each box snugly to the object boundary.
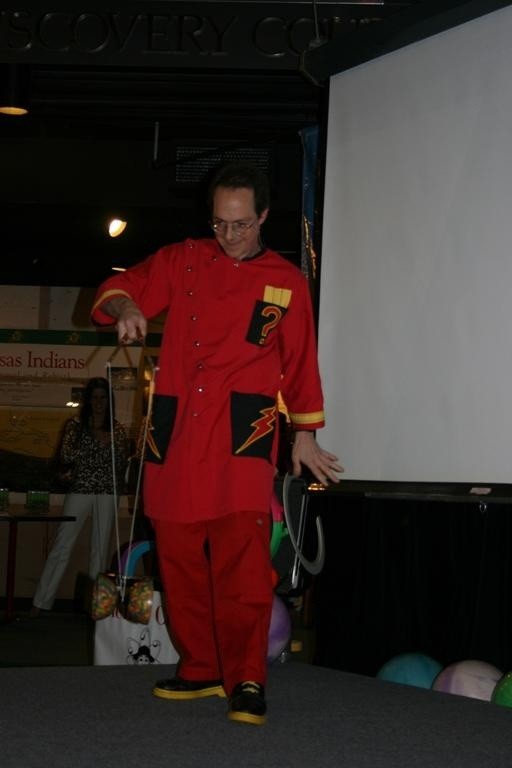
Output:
[0,94,27,116]
[105,210,128,240]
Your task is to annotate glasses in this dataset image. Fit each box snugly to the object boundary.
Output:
[209,217,258,234]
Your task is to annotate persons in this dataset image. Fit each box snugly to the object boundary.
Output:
[83,158,344,726]
[27,375,129,608]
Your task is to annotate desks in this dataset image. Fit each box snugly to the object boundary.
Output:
[0,505,76,613]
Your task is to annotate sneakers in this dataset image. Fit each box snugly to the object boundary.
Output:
[152,674,224,701]
[227,677,271,725]
